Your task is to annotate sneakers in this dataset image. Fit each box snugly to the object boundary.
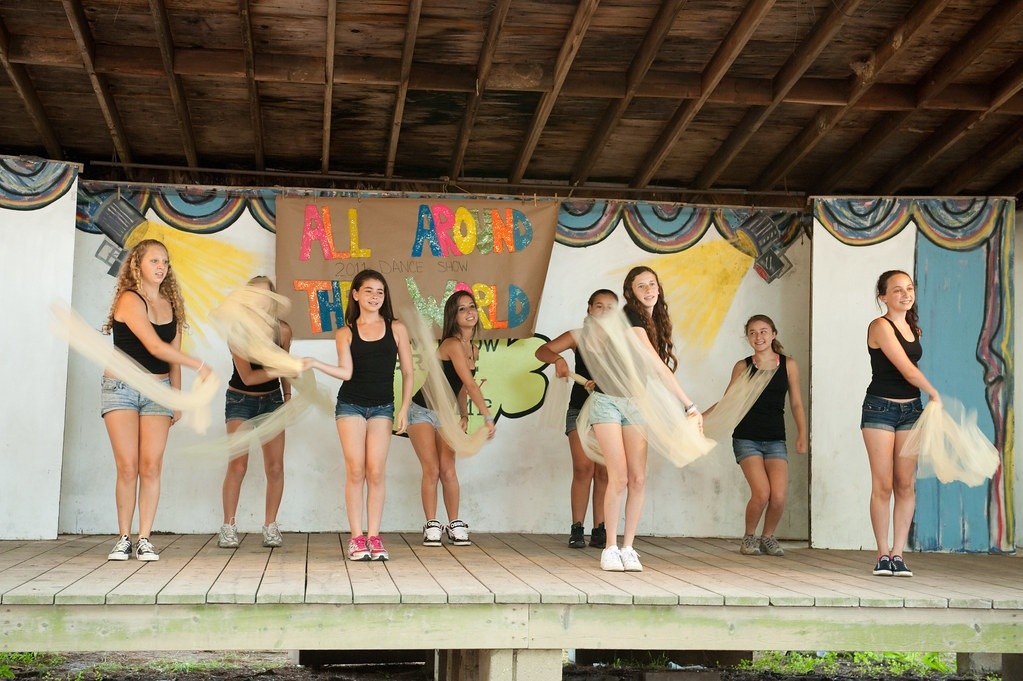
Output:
[568,522,585,548]
[108,535,132,561]
[759,534,785,555]
[873,554,893,576]
[133,538,159,561]
[368,536,388,561]
[348,536,372,562]
[262,522,284,547]
[620,545,643,571]
[422,519,444,546]
[218,517,239,549]
[600,543,625,572]
[446,519,471,546]
[589,524,606,548]
[738,534,760,555]
[890,554,913,576]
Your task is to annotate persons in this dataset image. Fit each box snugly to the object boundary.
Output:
[302,269,414,561]
[100,239,215,562]
[217,276,301,547]
[587,266,704,570]
[408,289,497,546]
[702,315,809,557]
[860,270,944,578]
[535,290,620,547]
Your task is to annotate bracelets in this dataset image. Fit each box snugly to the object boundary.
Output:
[197,361,204,372]
[685,404,697,413]
[461,415,469,422]
[484,415,494,420]
[555,357,563,362]
